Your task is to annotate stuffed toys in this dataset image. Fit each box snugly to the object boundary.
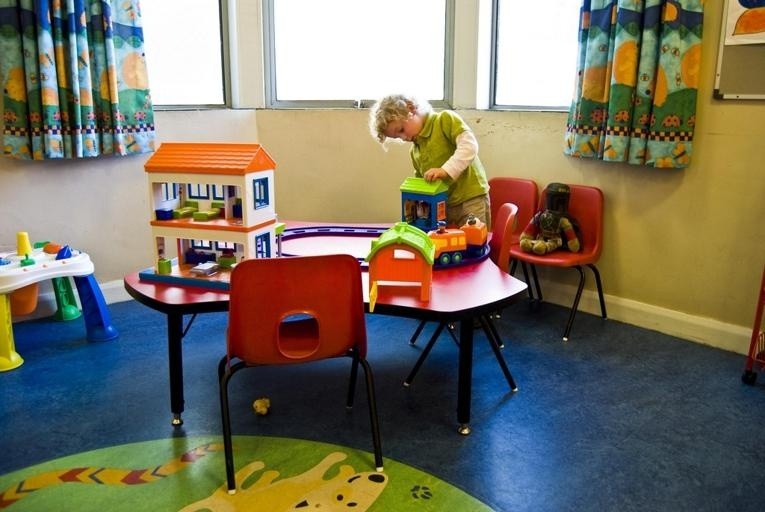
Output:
[519,183,580,255]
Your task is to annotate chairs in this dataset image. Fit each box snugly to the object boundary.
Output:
[496,183,608,343]
[485,178,543,321]
[401,201,520,393]
[217,253,384,498]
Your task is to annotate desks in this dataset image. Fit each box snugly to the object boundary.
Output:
[124,224,529,435]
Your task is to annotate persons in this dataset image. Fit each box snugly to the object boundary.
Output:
[369,95,491,232]
